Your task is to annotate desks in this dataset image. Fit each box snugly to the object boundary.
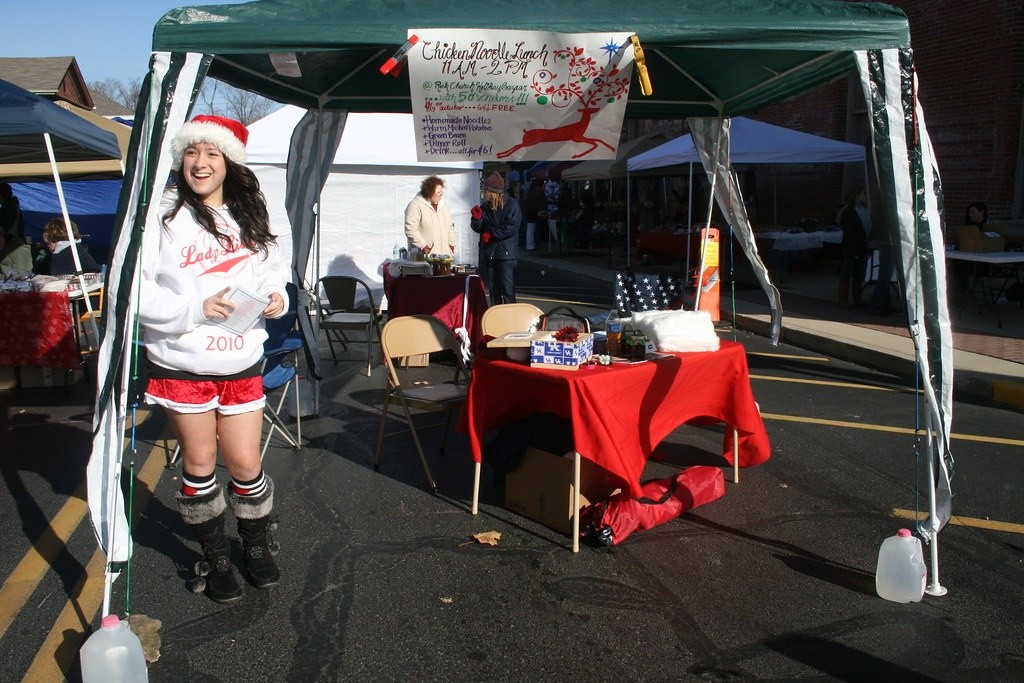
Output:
[0,282,106,398]
[471,341,745,552]
[949,250,1024,328]
[548,221,603,258]
[383,264,488,366]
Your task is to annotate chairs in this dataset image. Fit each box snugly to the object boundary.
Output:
[313,275,382,376]
[76,287,104,355]
[481,303,544,338]
[373,314,472,493]
[168,282,303,470]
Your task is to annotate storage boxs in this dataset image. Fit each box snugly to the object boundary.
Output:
[954,223,1008,253]
[504,446,622,535]
[398,353,430,367]
[531,332,594,371]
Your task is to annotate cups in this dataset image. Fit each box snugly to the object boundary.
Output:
[433,261,450,275]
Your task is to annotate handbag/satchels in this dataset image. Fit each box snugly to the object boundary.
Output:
[539,306,590,334]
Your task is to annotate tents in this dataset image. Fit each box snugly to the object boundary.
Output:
[559,132,767,270]
[509,156,571,257]
[0,78,136,348]
[626,115,871,277]
[86,0,954,629]
[243,104,482,316]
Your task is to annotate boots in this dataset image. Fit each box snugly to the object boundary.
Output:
[226,473,281,588]
[176,478,242,605]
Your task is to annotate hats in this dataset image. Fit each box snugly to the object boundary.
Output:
[171,115,248,169]
[483,171,504,194]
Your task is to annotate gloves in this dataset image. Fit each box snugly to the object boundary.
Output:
[481,232,491,243]
[471,205,483,220]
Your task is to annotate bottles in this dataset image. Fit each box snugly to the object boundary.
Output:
[393,242,400,260]
[875,529,927,604]
[605,310,622,357]
[80,615,149,683]
[399,247,454,266]
[622,325,646,359]
[288,375,314,417]
[102,264,107,273]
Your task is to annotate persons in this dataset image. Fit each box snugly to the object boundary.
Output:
[839,183,873,309]
[509,180,548,251]
[37,218,100,318]
[405,176,455,263]
[0,224,33,278]
[958,203,998,300]
[470,170,522,308]
[0,182,24,240]
[134,115,290,602]
